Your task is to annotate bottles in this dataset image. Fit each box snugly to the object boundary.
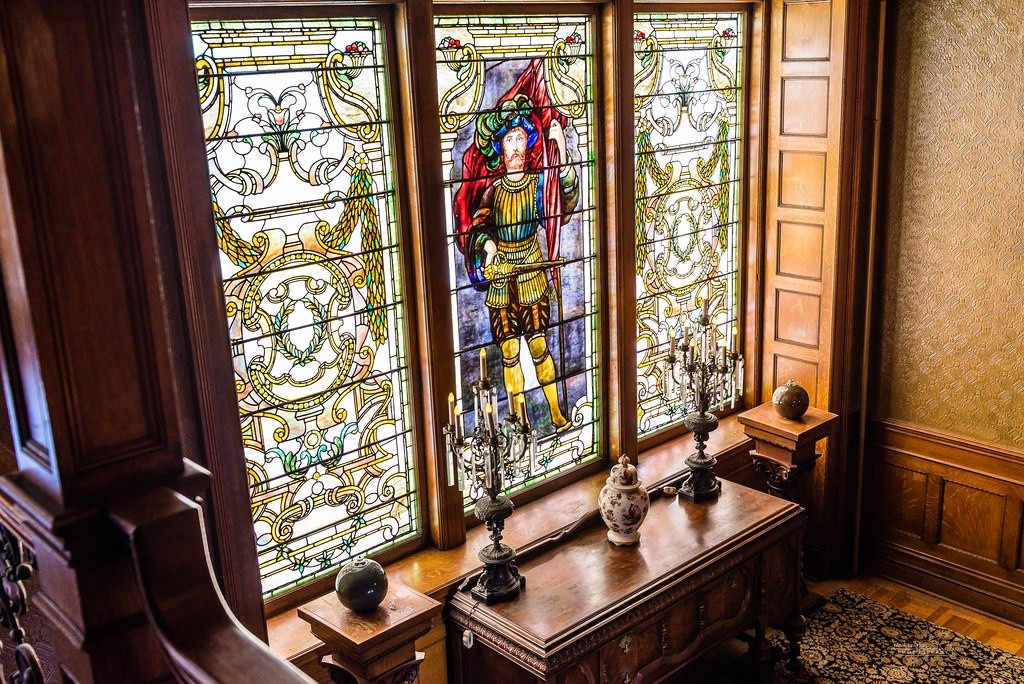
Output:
[599,456,649,545]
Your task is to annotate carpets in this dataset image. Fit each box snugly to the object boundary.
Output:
[707,588,1024,684]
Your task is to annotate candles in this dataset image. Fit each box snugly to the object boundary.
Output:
[449,347,528,440]
[670,299,738,369]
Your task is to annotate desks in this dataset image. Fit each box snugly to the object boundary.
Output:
[444,477,806,684]
